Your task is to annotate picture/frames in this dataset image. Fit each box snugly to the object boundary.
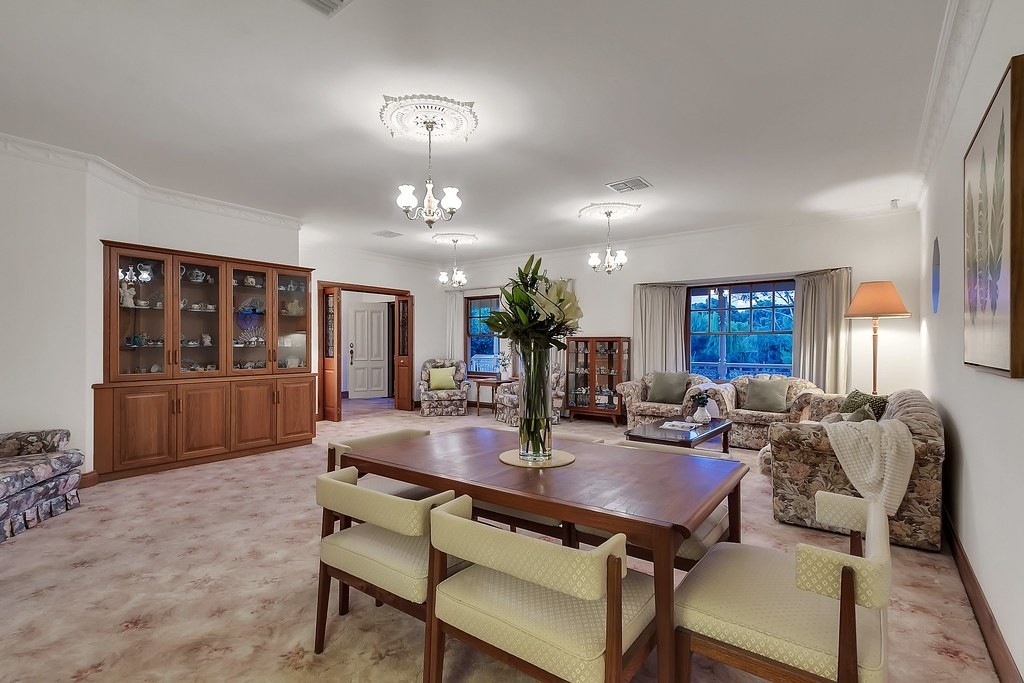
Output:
[963,54,1024,381]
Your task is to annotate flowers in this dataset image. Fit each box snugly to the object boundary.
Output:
[492,350,513,372]
[482,253,583,456]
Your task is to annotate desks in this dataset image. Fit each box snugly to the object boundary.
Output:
[338,422,752,683]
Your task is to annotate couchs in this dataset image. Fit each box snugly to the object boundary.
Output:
[415,358,472,417]
[768,389,947,553]
[708,374,825,452]
[0,428,88,543]
[493,359,566,427]
[615,372,716,430]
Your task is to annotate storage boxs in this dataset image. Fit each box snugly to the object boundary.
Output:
[496,373,501,381]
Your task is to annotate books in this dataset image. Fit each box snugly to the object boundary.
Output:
[658,421,703,431]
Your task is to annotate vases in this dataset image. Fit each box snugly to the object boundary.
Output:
[500,366,509,381]
[517,344,554,462]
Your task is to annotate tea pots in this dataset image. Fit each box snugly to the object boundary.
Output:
[187,267,205,282]
[160,264,186,280]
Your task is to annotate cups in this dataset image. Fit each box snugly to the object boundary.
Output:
[205,304,216,310]
[191,304,202,310]
[180,299,188,308]
[137,300,149,306]
[157,302,163,307]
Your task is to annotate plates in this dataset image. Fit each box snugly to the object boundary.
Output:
[189,310,201,312]
[203,310,216,312]
[153,307,163,309]
[134,306,150,309]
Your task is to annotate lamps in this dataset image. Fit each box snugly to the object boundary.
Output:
[578,202,641,276]
[379,93,478,231]
[431,233,479,288]
[844,280,913,397]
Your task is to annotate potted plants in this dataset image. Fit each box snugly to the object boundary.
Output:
[690,390,712,425]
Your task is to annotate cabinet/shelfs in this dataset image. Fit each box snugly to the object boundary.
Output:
[111,376,232,481]
[565,336,631,429]
[232,372,317,459]
[100,238,316,384]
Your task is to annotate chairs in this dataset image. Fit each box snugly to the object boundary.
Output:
[568,440,732,572]
[311,466,475,683]
[327,428,443,618]
[428,494,659,683]
[472,431,605,547]
[672,488,894,683]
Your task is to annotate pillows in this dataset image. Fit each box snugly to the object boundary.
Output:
[837,389,890,421]
[646,370,690,405]
[741,378,791,413]
[846,402,878,422]
[819,412,843,424]
[428,366,457,391]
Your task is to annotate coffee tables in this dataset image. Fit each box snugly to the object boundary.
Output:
[474,379,516,419]
[624,415,733,454]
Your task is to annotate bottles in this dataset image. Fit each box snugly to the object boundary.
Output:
[244,276,255,286]
